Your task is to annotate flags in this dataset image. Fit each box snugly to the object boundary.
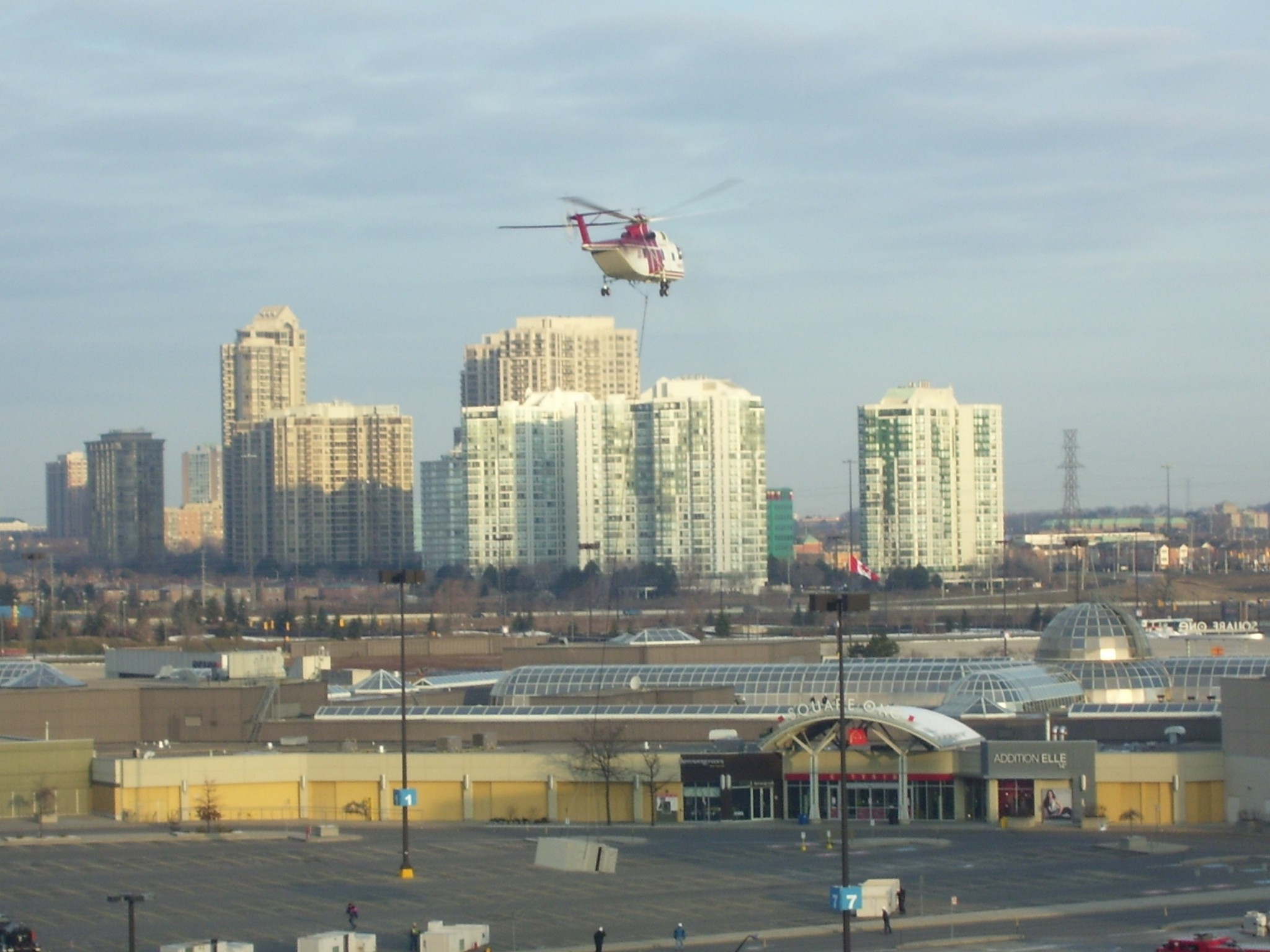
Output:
[849,555,880,583]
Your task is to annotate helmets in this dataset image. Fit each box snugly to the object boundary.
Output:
[599,927,603,931]
[678,922,682,927]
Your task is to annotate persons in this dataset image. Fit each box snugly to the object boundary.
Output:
[882,906,892,935]
[347,903,359,927]
[409,921,419,952]
[896,886,906,914]
[593,926,606,952]
[658,797,672,814]
[11,922,32,946]
[1043,789,1073,817]
[673,923,686,951]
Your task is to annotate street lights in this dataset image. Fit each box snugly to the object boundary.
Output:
[107,891,146,952]
[579,542,600,636]
[377,566,426,879]
[809,590,872,952]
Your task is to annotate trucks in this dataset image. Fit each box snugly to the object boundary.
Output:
[1156,938,1270,952]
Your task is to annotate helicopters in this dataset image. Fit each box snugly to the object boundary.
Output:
[495,179,742,297]
